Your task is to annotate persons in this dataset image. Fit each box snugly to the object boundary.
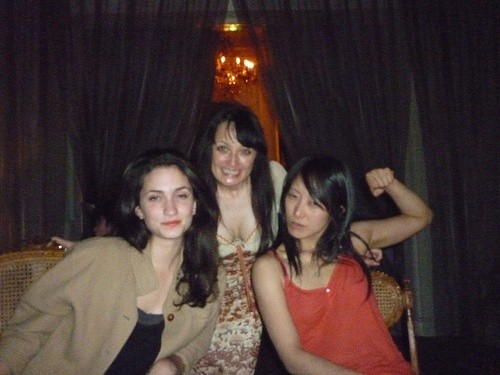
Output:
[52,96,382,375]
[251,155,433,375]
[0,146,226,375]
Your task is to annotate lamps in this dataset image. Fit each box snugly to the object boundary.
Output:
[210,24,262,95]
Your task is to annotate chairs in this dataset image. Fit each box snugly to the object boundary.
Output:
[0,249,65,330]
[369,272,420,375]
